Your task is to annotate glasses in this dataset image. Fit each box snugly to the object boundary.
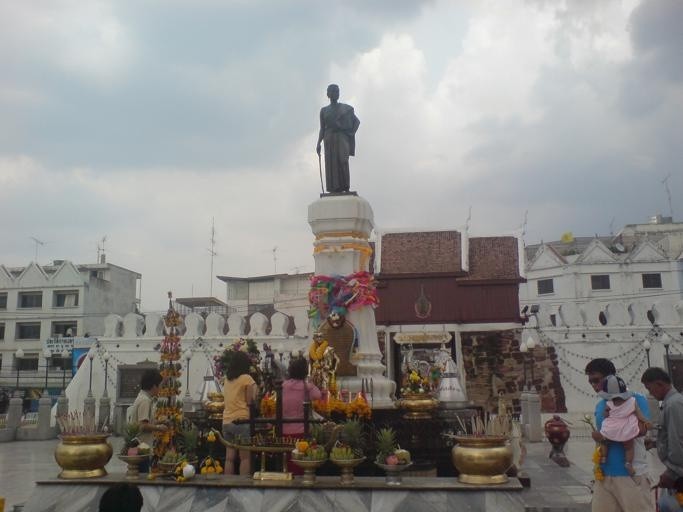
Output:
[588,376,604,384]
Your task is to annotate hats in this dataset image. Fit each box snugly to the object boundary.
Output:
[598,375,630,400]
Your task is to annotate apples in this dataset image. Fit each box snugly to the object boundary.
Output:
[386,455,399,465]
[128,448,138,456]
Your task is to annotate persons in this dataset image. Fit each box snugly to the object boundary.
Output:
[127,369,169,471]
[589,374,653,475]
[642,363,683,511]
[279,354,321,471]
[220,350,260,475]
[314,83,361,193]
[96,481,143,512]
[581,356,657,511]
[303,330,344,396]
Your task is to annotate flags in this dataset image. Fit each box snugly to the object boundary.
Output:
[560,232,574,244]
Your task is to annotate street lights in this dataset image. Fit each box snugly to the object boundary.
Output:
[102,351,109,389]
[60,348,70,391]
[184,348,192,389]
[42,346,52,389]
[643,339,651,369]
[660,333,673,386]
[277,342,283,366]
[520,342,528,391]
[13,346,23,388]
[526,335,536,390]
[290,346,298,361]
[87,349,95,390]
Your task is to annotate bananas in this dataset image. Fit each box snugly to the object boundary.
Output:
[330,447,354,460]
[307,446,327,461]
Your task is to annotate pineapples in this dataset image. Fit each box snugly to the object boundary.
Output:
[341,419,365,459]
[374,428,397,463]
[120,424,144,456]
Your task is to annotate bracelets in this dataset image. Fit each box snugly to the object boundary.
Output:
[156,424,160,431]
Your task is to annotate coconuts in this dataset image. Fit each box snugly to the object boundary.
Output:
[136,443,150,455]
[395,450,411,465]
[292,449,305,460]
